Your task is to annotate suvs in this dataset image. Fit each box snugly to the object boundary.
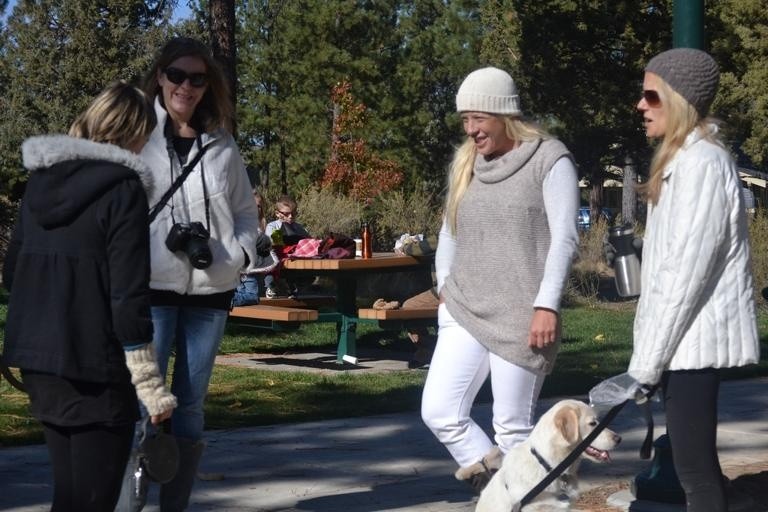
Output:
[578,206,613,234]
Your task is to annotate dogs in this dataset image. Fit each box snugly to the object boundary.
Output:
[475,398,623,512]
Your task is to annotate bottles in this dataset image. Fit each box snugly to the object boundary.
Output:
[361,221,373,258]
[606,222,642,298]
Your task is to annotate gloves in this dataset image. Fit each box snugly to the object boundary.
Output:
[600,233,643,268]
[627,381,663,406]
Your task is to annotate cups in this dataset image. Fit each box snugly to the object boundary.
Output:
[352,238,364,257]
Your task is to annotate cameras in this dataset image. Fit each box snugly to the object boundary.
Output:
[165,222,212,269]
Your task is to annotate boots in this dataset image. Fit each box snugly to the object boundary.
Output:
[160,438,207,511]
[453,443,506,497]
[114,449,154,512]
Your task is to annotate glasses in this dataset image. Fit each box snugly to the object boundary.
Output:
[156,62,210,89]
[639,89,662,110]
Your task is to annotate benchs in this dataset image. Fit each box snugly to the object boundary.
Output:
[357,307,439,321]
[229,304,316,322]
[258,294,337,309]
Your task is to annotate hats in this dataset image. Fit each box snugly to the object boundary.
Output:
[453,66,523,120]
[649,47,721,120]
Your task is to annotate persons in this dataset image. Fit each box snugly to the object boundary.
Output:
[416,64,582,495]
[0,78,180,512]
[227,189,280,313]
[402,235,438,353]
[623,42,763,511]
[128,34,266,511]
[264,193,319,302]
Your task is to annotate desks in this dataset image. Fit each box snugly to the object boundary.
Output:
[282,249,433,365]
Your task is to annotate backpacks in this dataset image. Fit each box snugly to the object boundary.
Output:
[286,232,357,262]
